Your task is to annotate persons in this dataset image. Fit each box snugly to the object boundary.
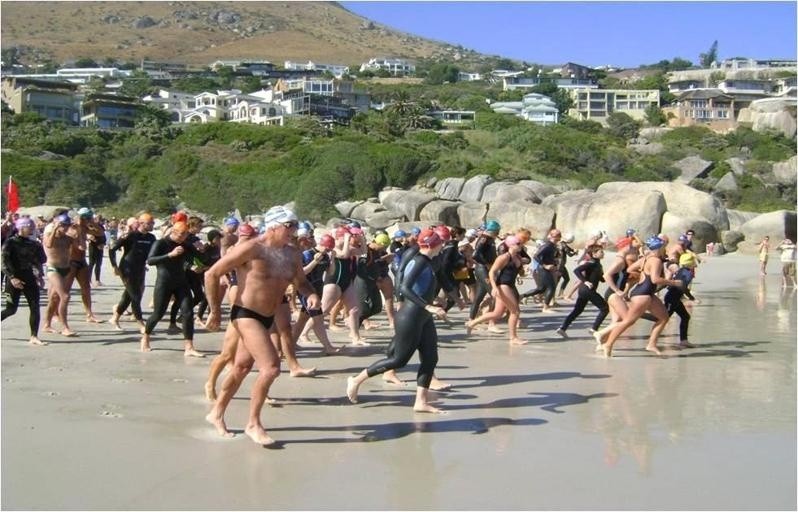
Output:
[205,206,320,443]
[776,238,797,288]
[346,231,453,413]
[428,220,699,360]
[1,208,423,378]
[759,236,769,276]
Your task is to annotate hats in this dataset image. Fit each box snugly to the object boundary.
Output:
[15,207,254,238]
[299,220,530,250]
[265,206,297,227]
[537,229,696,270]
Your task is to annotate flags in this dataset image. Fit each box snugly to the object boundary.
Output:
[6,179,19,213]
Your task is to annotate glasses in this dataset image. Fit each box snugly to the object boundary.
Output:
[274,221,298,230]
